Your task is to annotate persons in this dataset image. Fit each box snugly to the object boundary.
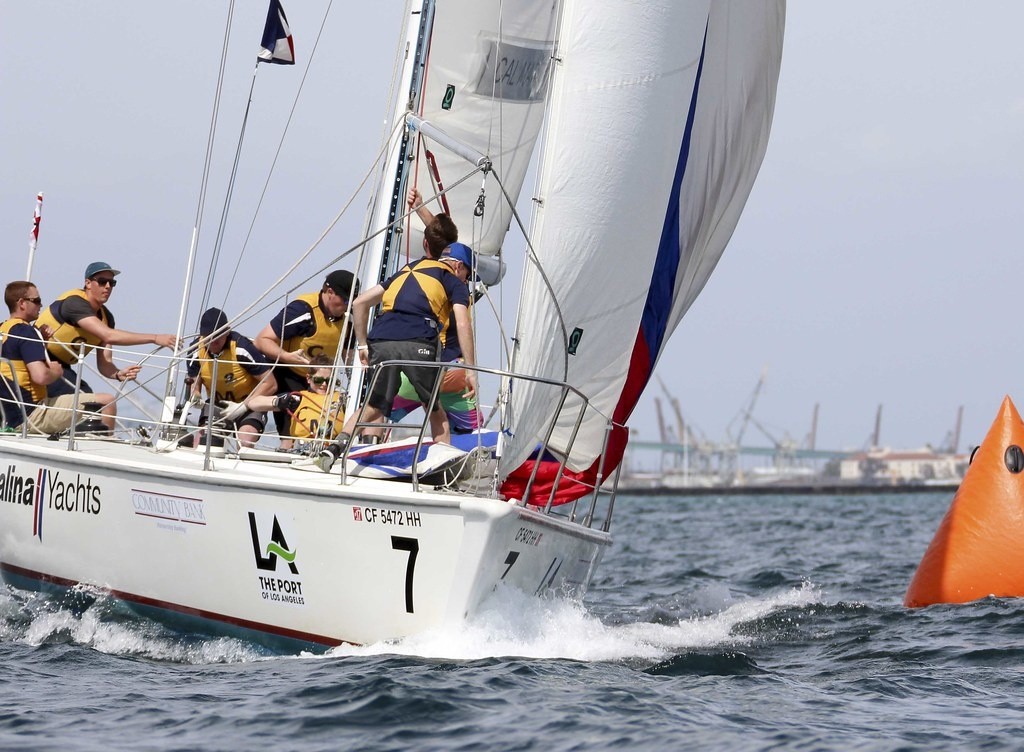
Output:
[0,281,118,436]
[312,242,481,495]
[33,262,183,399]
[358,188,485,445]
[188,270,361,453]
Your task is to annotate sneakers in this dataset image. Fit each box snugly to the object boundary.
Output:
[314,444,340,473]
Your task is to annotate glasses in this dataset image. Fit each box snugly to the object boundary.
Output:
[463,264,471,280]
[310,375,330,385]
[90,278,116,288]
[17,297,41,305]
[343,296,350,305]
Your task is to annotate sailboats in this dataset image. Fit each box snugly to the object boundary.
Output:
[0,0,791,660]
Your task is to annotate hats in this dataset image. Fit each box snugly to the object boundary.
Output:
[200,308,228,335]
[327,270,360,304]
[441,243,481,282]
[85,262,120,279]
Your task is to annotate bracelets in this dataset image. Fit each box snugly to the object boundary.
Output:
[357,345,369,350]
[154,334,158,344]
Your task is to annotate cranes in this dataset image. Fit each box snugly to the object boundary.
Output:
[944,404,965,455]
[854,403,905,487]
[648,364,825,486]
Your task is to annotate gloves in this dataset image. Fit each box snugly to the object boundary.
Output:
[274,392,302,412]
[191,394,206,409]
[219,400,248,422]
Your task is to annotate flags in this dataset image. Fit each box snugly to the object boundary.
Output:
[116,370,122,383]
[256,0,296,65]
[30,195,43,249]
